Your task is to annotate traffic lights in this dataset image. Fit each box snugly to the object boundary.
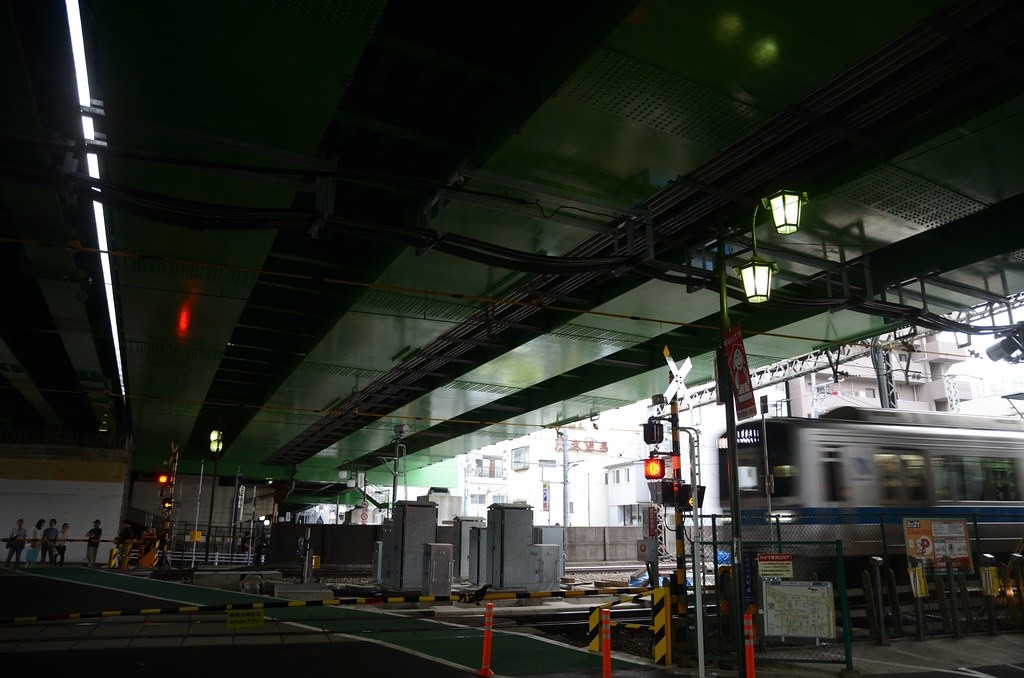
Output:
[679,484,694,511]
[157,473,172,486]
[163,498,172,509]
[644,459,665,479]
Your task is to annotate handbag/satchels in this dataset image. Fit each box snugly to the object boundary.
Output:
[25,548,38,562]
[4,535,17,548]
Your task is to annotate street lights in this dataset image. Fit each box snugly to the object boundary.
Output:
[204,428,225,566]
[713,187,812,665]
[376,423,410,519]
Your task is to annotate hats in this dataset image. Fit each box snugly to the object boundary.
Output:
[122,519,130,525]
[92,519,100,523]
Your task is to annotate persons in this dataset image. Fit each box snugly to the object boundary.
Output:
[56,523,71,568]
[40,519,58,569]
[115,520,132,567]
[242,532,253,564]
[4,519,27,570]
[25,519,46,569]
[85,519,102,568]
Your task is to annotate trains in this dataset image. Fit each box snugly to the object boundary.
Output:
[718,406,1024,613]
